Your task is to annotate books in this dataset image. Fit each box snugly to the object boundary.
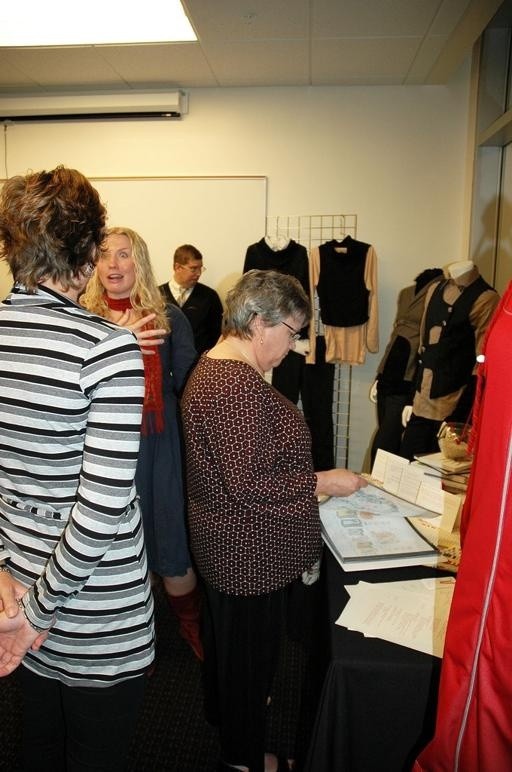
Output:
[411,451,473,494]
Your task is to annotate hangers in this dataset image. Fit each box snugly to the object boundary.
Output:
[334,212,345,242]
[271,216,288,246]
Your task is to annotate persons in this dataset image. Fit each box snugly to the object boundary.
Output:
[367,259,499,475]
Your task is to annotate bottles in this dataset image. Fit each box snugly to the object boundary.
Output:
[438,421,476,460]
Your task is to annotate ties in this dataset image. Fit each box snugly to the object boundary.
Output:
[176,287,188,306]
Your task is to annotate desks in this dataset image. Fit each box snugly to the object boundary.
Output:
[322,538,456,770]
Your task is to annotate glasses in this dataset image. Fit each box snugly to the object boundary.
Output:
[282,319,302,346]
[181,264,208,275]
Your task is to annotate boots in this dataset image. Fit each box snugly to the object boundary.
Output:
[166,583,205,663]
